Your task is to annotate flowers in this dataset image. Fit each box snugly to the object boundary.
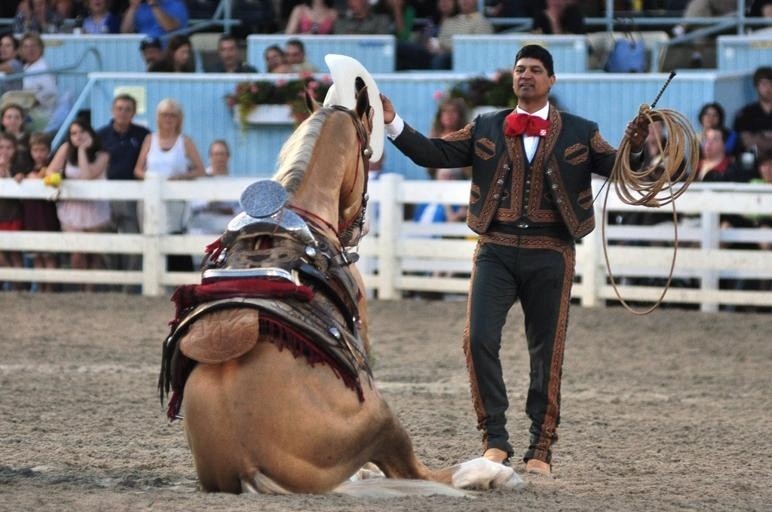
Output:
[221,69,332,132]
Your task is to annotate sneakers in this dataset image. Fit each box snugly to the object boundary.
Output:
[482,447,508,465]
[525,458,552,477]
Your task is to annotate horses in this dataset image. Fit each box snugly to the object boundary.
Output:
[182,74,523,501]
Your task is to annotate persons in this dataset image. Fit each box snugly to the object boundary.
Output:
[608,65,772,316]
[0,1,339,292]
[336,2,492,68]
[376,44,650,481]
[532,0,601,71]
[409,98,477,303]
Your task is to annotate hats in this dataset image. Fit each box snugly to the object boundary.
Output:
[322,54,385,164]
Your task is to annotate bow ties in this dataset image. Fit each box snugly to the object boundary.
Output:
[504,113,549,136]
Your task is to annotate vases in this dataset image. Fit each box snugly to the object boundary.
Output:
[233,104,296,128]
